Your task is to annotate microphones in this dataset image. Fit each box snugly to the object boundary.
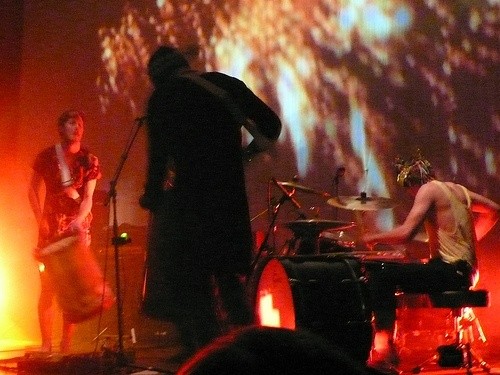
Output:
[272,177,300,208]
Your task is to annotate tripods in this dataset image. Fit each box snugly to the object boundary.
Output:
[84,111,175,375]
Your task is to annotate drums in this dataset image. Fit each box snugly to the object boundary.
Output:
[252,252,375,365]
[33,230,117,324]
[285,229,357,256]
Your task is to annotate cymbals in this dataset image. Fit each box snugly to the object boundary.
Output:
[325,194,401,211]
[270,181,330,196]
[278,218,357,232]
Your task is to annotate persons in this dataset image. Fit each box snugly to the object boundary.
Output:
[360,157,500,364]
[28,109,100,353]
[138,45,282,363]
